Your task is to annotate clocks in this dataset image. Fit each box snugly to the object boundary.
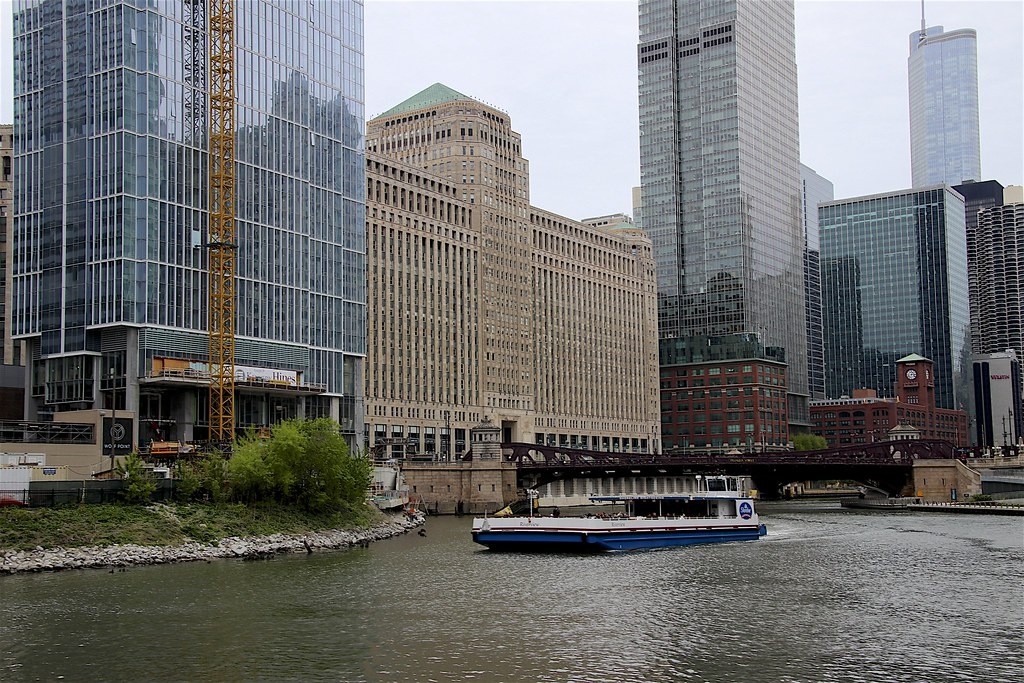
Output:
[925,369,929,380]
[905,369,917,380]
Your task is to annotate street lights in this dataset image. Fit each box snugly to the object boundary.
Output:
[759,430,766,453]
[748,432,754,454]
[1002,415,1007,446]
[1008,407,1014,446]
[448,414,451,461]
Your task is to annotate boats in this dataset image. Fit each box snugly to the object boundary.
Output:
[471,474,767,553]
